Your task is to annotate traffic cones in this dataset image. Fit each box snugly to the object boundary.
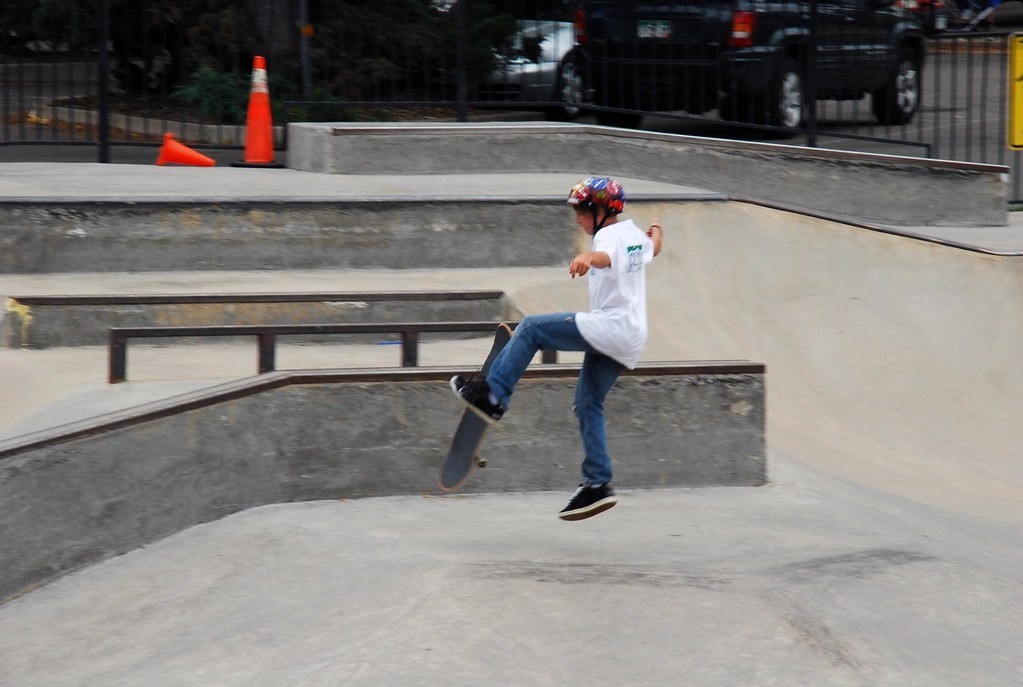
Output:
[154,131,218,167]
[228,54,287,169]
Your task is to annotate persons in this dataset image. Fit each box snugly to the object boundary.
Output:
[450,175,662,521]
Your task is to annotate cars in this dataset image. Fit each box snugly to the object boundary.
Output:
[427,18,595,121]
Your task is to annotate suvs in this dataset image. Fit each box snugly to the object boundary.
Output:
[572,0,927,141]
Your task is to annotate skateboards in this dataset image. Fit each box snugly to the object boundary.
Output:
[440,322,517,491]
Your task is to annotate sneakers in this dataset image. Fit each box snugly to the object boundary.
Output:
[557,481,617,519]
[448,374,510,426]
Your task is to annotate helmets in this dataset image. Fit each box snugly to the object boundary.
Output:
[568,175,638,217]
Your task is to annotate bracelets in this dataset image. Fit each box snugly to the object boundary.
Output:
[651,225,659,228]
